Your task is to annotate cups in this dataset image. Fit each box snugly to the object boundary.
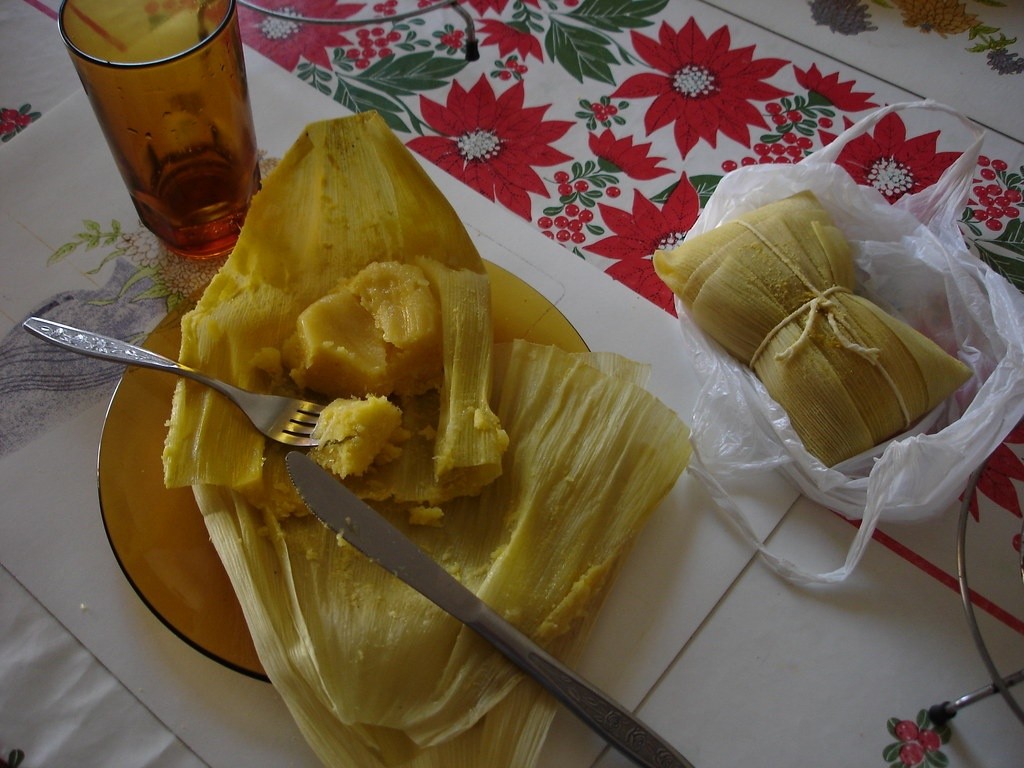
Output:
[56,0,261,260]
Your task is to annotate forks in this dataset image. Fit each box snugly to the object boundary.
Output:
[22,317,326,447]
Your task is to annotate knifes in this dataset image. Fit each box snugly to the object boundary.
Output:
[285,451,694,768]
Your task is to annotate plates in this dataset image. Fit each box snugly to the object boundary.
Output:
[96,256,593,682]
